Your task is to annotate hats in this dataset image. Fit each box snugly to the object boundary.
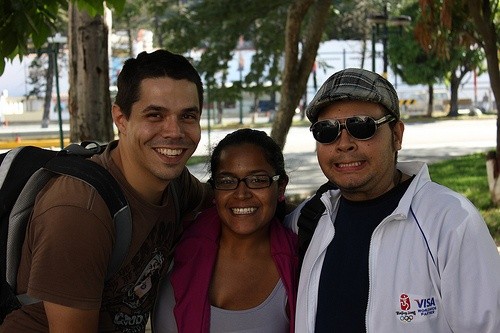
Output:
[306,67,400,122]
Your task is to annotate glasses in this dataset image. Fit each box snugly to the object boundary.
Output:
[310,114,395,143]
[211,174,282,190]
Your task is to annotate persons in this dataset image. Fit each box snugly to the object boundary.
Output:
[0,50,297,333]
[284,68,499,332]
[150,128,298,333]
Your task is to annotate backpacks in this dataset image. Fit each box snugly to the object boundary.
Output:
[0,140,201,324]
